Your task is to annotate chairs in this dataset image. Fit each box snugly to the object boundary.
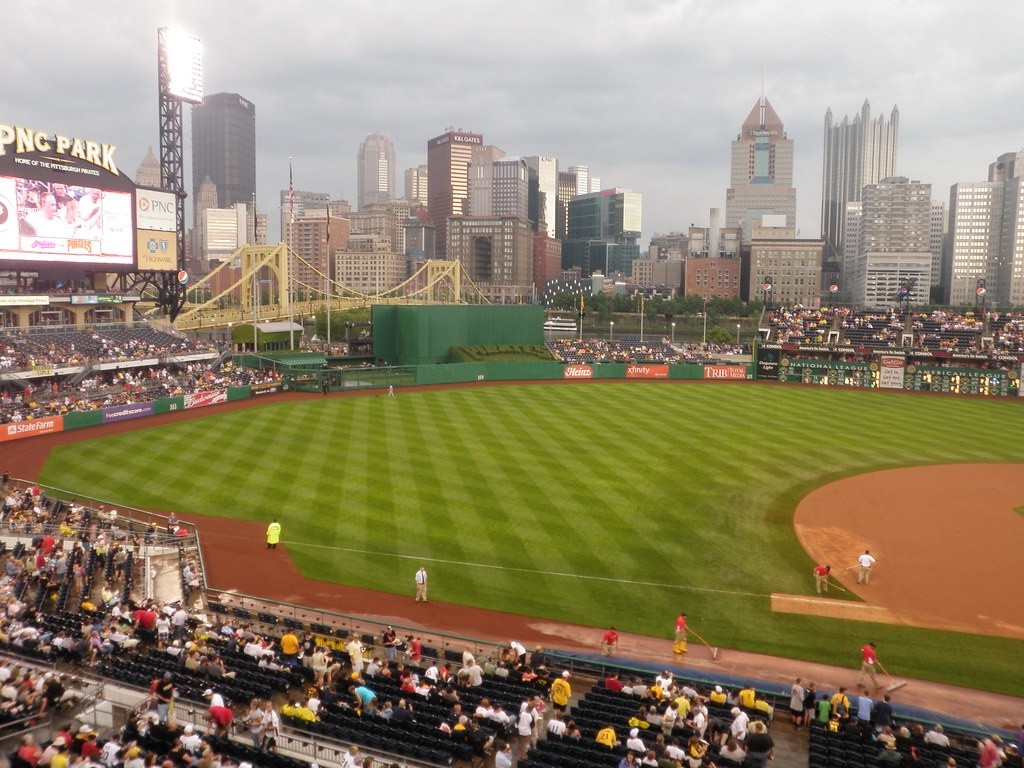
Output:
[21,327,194,364]
[545,341,715,363]
[768,311,1005,351]
[0,496,1024,768]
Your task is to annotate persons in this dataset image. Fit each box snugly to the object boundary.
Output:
[18,184,101,240]
[414,567,429,603]
[673,613,688,653]
[856,641,884,690]
[813,564,830,593]
[0,284,1023,768]
[600,626,618,656]
[857,550,875,584]
[267,518,281,550]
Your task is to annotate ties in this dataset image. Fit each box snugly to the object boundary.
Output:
[422,572,425,584]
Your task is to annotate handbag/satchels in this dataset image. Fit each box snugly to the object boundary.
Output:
[837,695,848,716]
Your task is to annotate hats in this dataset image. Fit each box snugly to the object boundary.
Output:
[351,672,359,679]
[142,600,287,678]
[387,625,393,631]
[151,523,156,529]
[52,737,65,746]
[459,716,468,724]
[184,723,193,733]
[202,688,212,696]
[562,670,570,677]
[630,728,639,737]
[650,706,656,712]
[79,724,92,733]
[839,687,847,692]
[715,685,722,692]
[536,645,541,650]
[493,703,502,710]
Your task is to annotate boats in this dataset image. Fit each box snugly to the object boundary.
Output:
[543,317,578,331]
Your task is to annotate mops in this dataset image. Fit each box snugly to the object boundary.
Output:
[876,664,908,693]
[688,628,718,660]
